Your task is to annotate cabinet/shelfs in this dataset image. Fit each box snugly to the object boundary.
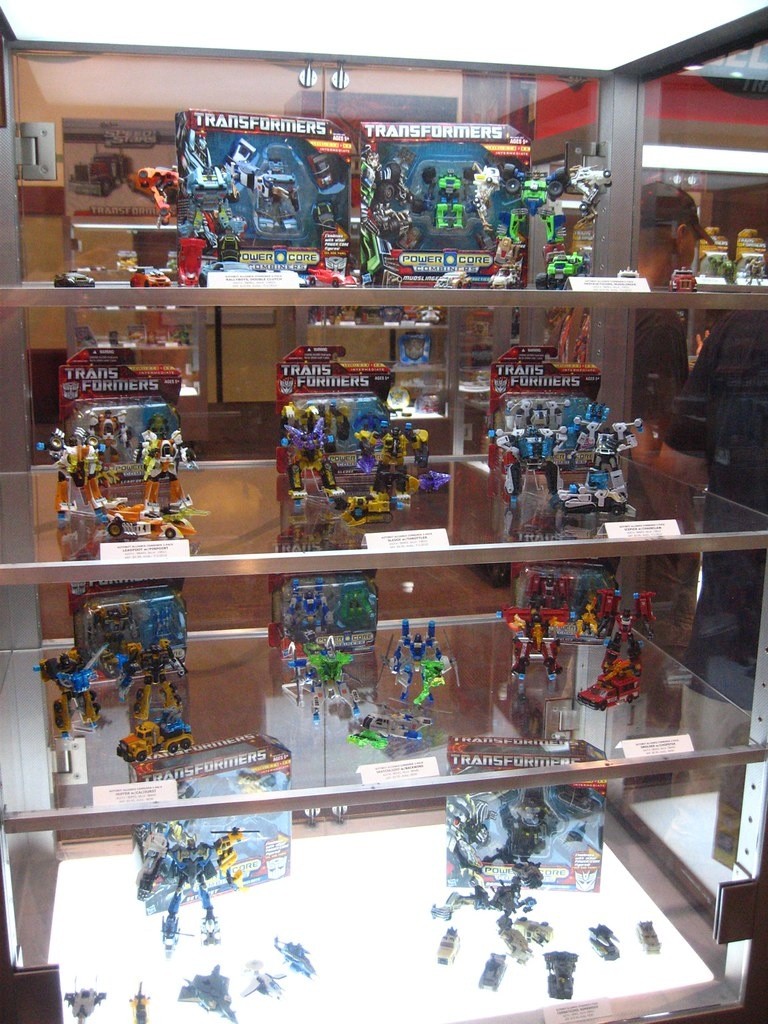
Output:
[1,8,768,1024]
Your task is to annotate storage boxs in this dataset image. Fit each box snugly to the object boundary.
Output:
[446,735,603,892]
[175,108,527,292]
[124,734,304,917]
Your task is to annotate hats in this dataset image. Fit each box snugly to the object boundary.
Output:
[637,176,716,246]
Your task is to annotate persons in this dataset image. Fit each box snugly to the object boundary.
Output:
[623,182,703,663]
[661,311,768,749]
